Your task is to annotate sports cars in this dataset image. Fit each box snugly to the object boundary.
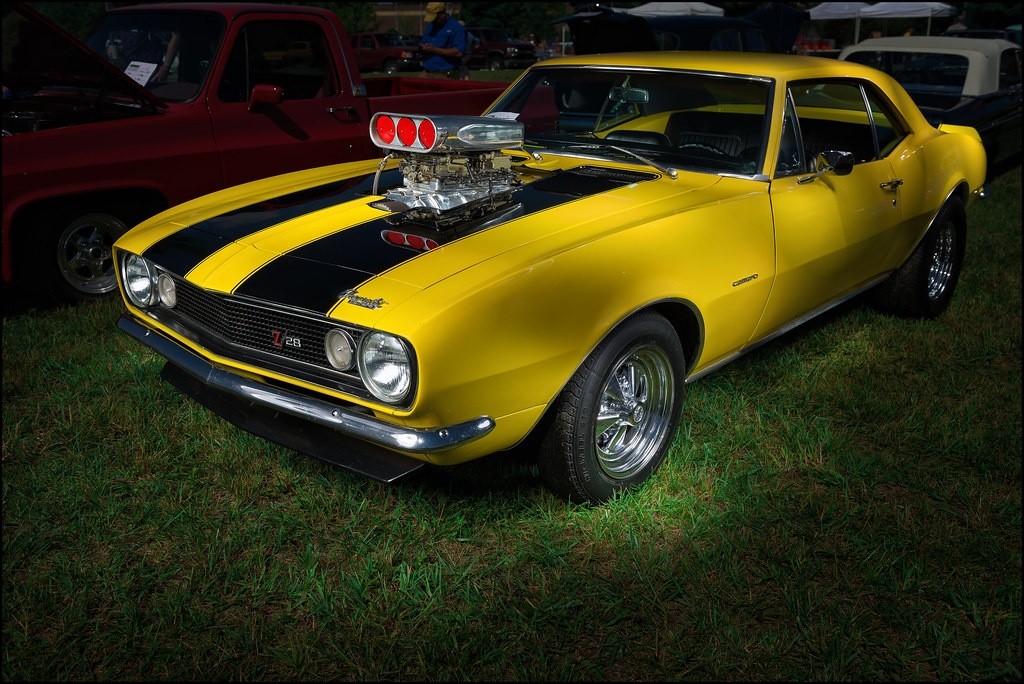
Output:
[110,51,987,508]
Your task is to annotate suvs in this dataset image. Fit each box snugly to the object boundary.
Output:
[464,26,537,71]
[348,32,419,74]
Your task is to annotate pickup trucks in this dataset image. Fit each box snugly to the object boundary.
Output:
[0,2,560,298]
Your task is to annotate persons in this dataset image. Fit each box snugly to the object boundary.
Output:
[902,25,917,36]
[869,24,883,38]
[418,1,466,79]
[946,11,967,31]
[117,24,182,84]
[457,20,480,79]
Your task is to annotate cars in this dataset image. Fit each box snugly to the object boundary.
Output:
[794,35,1024,179]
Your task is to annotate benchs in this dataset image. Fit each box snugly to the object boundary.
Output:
[660,109,895,175]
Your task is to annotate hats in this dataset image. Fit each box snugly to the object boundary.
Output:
[423,2,445,22]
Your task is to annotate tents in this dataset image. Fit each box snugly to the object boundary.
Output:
[803,0,957,46]
[559,2,726,56]
